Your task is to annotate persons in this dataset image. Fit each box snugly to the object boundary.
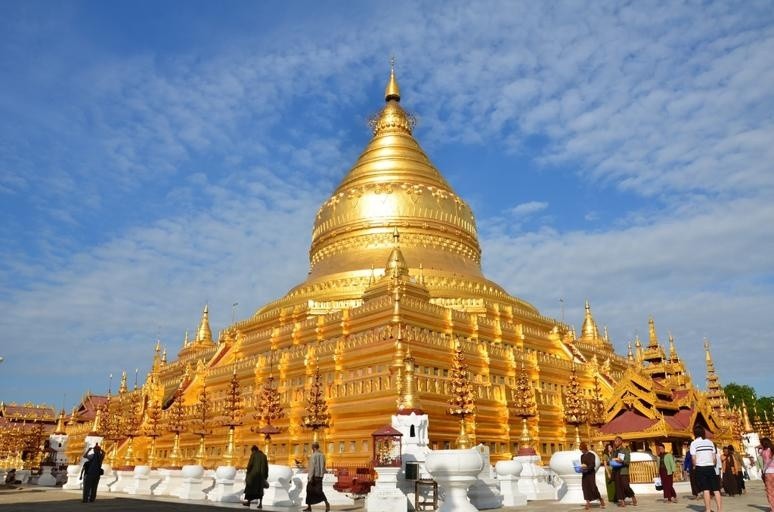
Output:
[657,423,774,512]
[6,469,16,484]
[79,442,106,504]
[303,441,331,512]
[579,436,638,509]
[243,445,269,508]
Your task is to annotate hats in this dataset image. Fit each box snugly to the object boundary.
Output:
[580,442,587,450]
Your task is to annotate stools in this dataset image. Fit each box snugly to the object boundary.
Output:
[415,480,437,512]
